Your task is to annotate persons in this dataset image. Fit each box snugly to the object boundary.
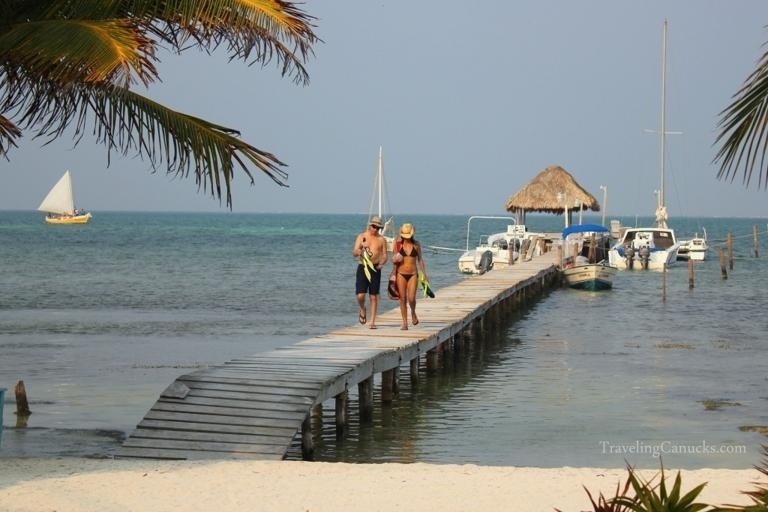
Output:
[391,223,429,332]
[351,217,388,328]
[48,207,85,219]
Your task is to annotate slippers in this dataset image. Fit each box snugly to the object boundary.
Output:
[369,324,376,329]
[359,307,367,324]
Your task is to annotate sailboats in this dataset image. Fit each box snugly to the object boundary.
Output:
[37,169,93,224]
[609,19,710,262]
[364,145,403,252]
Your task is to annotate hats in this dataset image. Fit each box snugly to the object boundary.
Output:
[368,216,384,228]
[399,223,414,240]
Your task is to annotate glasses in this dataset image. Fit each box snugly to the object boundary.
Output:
[369,224,381,230]
[365,247,373,257]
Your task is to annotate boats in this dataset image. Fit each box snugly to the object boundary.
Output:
[458,215,546,275]
[555,224,619,291]
[607,227,681,272]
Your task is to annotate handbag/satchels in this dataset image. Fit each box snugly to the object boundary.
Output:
[387,270,400,300]
